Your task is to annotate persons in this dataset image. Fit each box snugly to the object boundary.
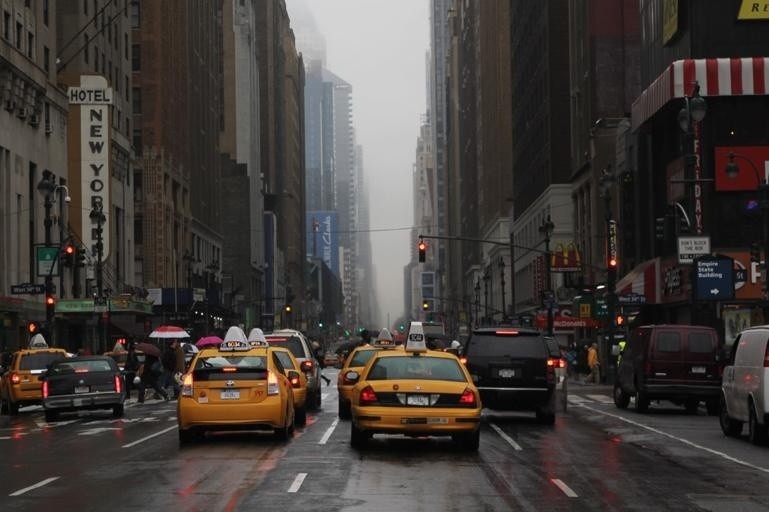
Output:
[124,341,140,399]
[564,340,626,384]
[154,337,181,399]
[171,343,186,400]
[334,327,468,365]
[310,340,330,386]
[137,354,168,403]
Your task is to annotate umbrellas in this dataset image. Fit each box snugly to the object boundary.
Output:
[134,344,162,358]
[195,336,223,346]
[148,325,191,340]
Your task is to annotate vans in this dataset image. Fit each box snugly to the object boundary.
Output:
[613,323,769,444]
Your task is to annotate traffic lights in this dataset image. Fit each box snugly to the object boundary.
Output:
[285,304,292,312]
[47,296,54,316]
[423,300,428,310]
[419,243,426,263]
[64,245,86,267]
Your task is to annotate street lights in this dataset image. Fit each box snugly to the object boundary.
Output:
[538,214,555,336]
[600,166,616,365]
[678,82,707,324]
[498,256,507,322]
[474,273,490,327]
[37,179,66,296]
[89,202,106,295]
